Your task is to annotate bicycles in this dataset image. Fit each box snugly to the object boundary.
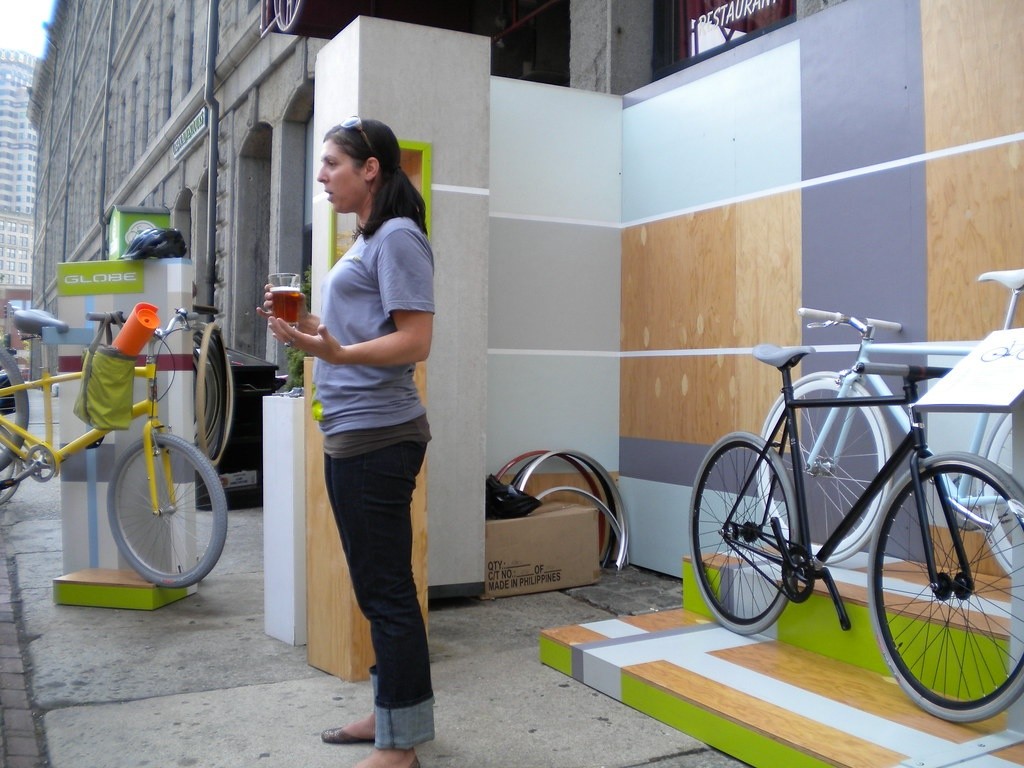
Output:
[688,339,1024,721]
[755,268,1024,575]
[1,305,228,588]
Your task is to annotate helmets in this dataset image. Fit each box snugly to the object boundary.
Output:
[121,228,188,259]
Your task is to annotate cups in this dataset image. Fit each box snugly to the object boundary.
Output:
[267,273,302,331]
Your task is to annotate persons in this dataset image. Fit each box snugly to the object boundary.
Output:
[256,119,435,768]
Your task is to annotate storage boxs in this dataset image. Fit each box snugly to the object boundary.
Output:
[485,499,600,599]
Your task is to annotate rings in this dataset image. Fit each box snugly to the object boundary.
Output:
[284,341,291,348]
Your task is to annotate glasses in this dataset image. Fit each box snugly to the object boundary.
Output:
[341,114,375,153]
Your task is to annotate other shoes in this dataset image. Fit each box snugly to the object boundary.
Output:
[321,725,376,744]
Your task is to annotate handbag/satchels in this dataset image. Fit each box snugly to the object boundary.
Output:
[72,311,137,431]
